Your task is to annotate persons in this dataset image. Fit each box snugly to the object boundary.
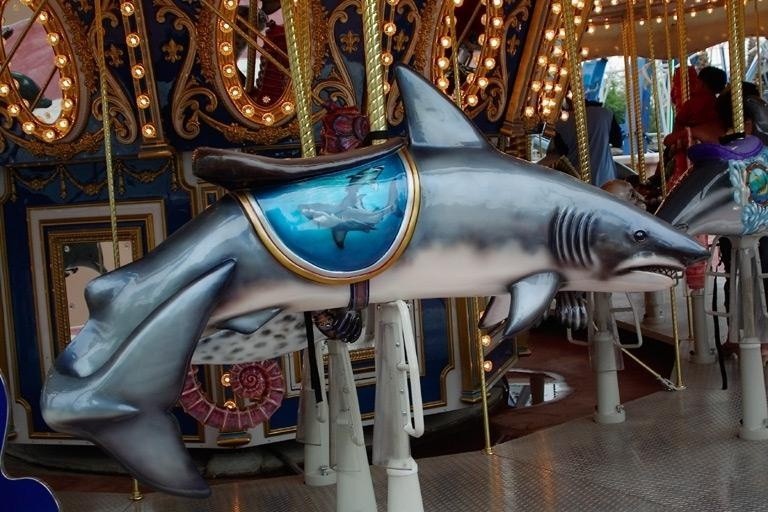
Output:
[663,66,768,367]
[547,95,623,188]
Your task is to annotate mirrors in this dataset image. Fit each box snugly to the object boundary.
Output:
[196,0,331,133]
[0,0,94,142]
[419,0,503,112]
